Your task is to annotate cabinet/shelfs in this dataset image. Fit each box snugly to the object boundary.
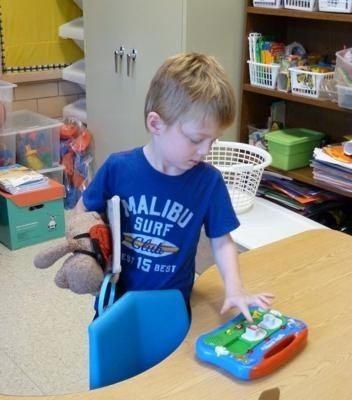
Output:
[83,1,246,180]
[236,1,352,197]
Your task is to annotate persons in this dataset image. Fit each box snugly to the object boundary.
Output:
[73,51,276,323]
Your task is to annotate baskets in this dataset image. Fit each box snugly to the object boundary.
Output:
[288,64,336,99]
[248,122,269,152]
[253,0,352,13]
[201,138,272,214]
[247,60,281,90]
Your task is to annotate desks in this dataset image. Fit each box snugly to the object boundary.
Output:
[0,228,352,400]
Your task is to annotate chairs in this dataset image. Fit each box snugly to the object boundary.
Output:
[88,289,190,390]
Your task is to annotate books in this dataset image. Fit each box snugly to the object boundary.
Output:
[309,144,352,193]
[242,169,343,218]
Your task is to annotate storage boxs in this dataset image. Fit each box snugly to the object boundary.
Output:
[0,81,67,251]
[265,127,326,172]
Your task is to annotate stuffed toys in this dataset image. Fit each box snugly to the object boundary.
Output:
[34,211,120,296]
